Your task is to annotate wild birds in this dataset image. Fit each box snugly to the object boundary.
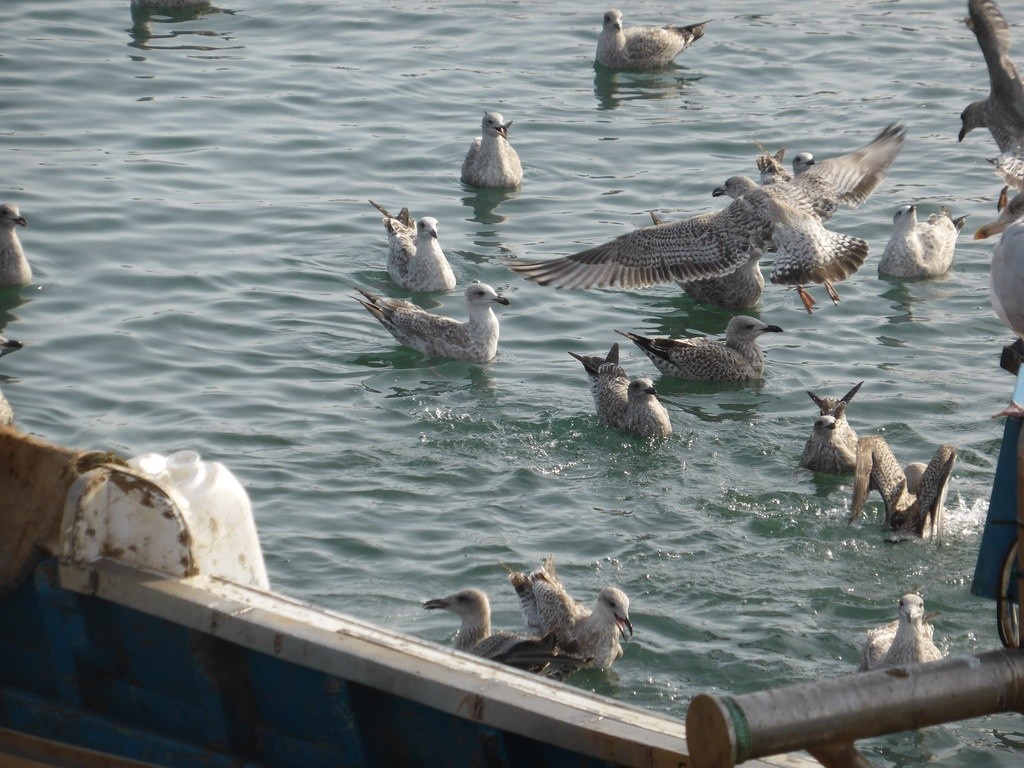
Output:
[498,118,910,313]
[460,111,523,189]
[875,205,972,279]
[0,336,24,360]
[865,593,943,672]
[847,435,958,543]
[565,343,673,440]
[597,10,715,70]
[800,380,865,475]
[958,0,1024,340]
[614,315,784,382]
[130,0,214,9]
[418,551,634,684]
[0,202,34,285]
[348,279,510,363]
[368,199,457,294]
